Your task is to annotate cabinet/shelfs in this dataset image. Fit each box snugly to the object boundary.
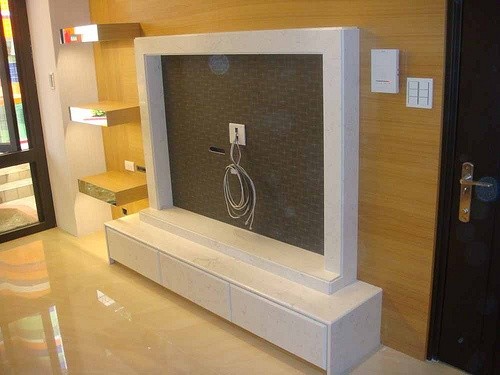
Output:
[104,212,382,375]
[58,22,148,207]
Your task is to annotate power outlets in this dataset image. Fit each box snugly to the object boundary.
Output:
[124,160,136,172]
